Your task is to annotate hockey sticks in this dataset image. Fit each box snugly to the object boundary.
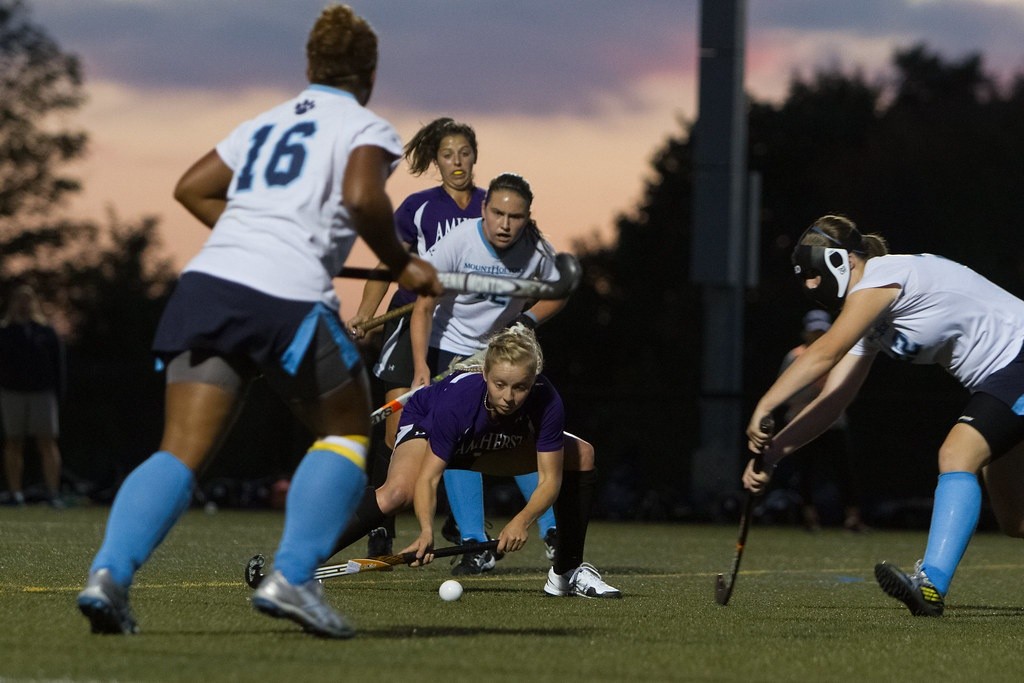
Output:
[345,300,416,336]
[717,416,775,607]
[369,365,450,426]
[245,540,499,589]
[335,252,583,299]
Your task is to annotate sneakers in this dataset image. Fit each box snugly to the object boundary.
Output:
[543,562,622,599]
[365,553,393,571]
[451,539,496,576]
[441,515,505,561]
[253,570,354,639]
[544,526,556,562]
[875,559,945,617]
[77,568,141,634]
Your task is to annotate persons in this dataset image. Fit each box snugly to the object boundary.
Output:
[0,286,70,510]
[77,3,450,638]
[322,116,621,600]
[743,216,1024,616]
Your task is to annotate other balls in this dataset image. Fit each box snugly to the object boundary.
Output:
[438,579,463,602]
